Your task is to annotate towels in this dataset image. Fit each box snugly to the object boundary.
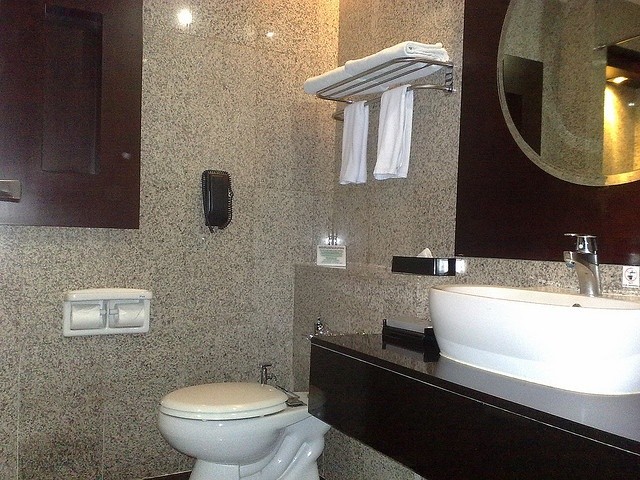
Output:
[336,99,370,188]
[302,41,452,98]
[371,83,416,182]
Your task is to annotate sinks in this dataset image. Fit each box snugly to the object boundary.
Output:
[427,284,639,396]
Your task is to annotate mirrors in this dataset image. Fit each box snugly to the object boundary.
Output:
[491,0,640,188]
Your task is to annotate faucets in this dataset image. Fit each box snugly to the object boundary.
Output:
[562,231,601,297]
[261,363,278,385]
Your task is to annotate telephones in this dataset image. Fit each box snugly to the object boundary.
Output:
[202,170,233,233]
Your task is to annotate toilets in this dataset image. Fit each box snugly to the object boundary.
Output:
[157,380,330,480]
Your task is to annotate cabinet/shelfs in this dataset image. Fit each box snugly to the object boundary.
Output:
[1,0,144,232]
